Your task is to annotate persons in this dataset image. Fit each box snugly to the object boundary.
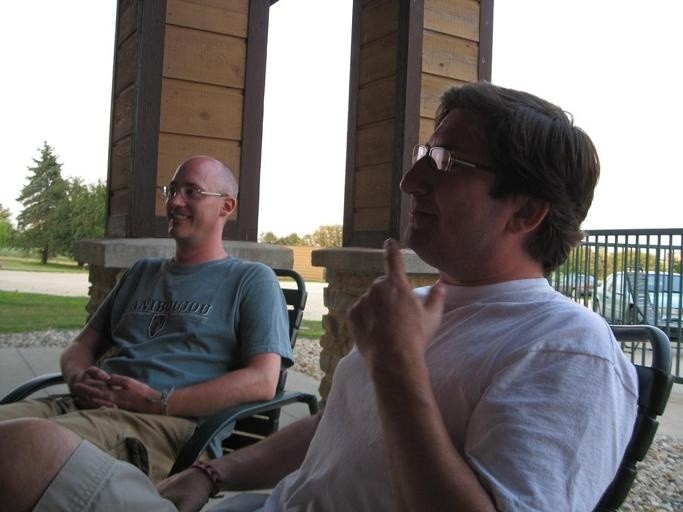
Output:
[0,80,639,511]
[0,154,294,490]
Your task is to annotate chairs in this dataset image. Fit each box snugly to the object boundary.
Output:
[0,268,309,458]
[165,325,673,511]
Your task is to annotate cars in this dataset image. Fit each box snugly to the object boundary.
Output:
[592,270,682,338]
[552,273,593,299]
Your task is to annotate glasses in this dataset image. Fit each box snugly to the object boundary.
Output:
[411,144,494,173]
[163,185,227,199]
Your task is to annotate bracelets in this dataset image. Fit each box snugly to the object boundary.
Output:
[193,459,224,498]
[159,383,169,414]
